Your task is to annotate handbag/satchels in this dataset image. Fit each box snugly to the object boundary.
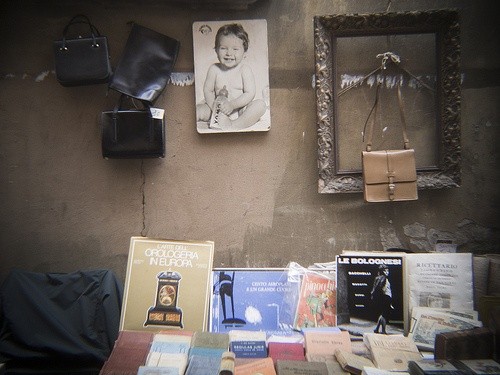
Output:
[360,51,419,202]
[106,21,181,106]
[100,93,166,160]
[0,268,124,375]
[52,13,112,87]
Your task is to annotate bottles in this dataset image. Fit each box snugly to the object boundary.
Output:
[209,85,228,129]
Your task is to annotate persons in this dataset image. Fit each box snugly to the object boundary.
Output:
[372,268,393,334]
[197,24,266,129]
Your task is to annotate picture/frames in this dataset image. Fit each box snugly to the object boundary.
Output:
[312,9,463,194]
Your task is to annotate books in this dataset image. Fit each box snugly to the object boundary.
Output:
[101,236,500,375]
